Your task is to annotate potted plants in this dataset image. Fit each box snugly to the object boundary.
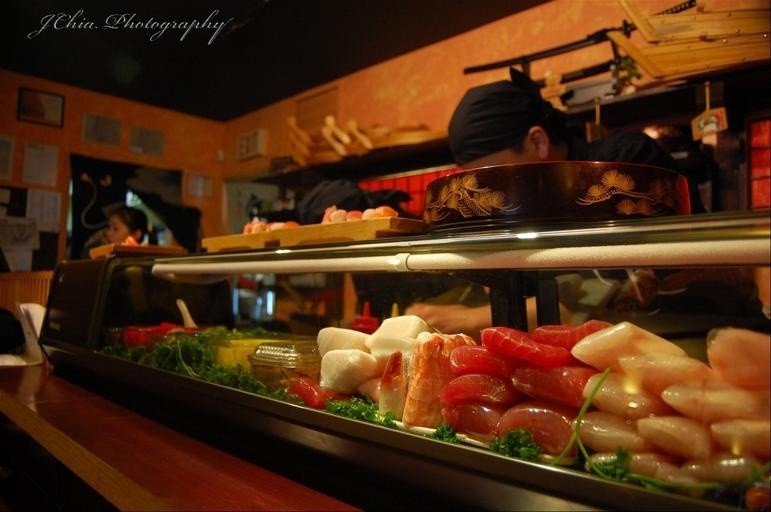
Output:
[17,86,65,128]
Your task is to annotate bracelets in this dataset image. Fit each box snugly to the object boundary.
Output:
[423,162,692,237]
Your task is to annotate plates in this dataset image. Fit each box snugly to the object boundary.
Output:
[37,206,771,512]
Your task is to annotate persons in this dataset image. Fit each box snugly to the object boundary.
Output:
[103,206,149,247]
[296,178,456,320]
[403,66,771,334]
